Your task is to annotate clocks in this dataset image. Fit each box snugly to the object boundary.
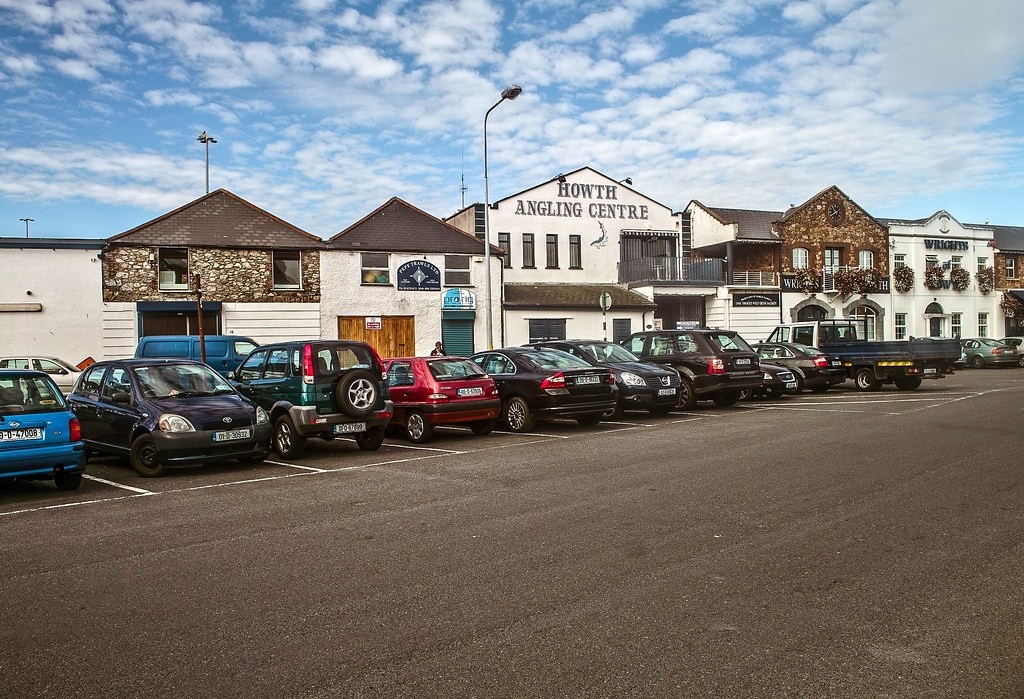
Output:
[824,199,846,227]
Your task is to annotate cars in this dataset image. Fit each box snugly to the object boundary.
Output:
[0,369,86,495]
[0,355,84,398]
[738,363,798,401]
[960,339,1020,369]
[64,357,276,478]
[520,340,682,422]
[999,336,1024,368]
[468,347,620,433]
[915,337,967,371]
[381,356,502,443]
[748,342,848,395]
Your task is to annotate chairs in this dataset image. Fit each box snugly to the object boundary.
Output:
[0,386,24,406]
[317,357,328,374]
[391,366,412,386]
[652,336,667,355]
[116,372,130,394]
[494,360,507,373]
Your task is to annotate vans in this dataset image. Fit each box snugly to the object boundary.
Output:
[134,333,297,381]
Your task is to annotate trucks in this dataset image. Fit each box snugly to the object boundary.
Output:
[766,321,961,392]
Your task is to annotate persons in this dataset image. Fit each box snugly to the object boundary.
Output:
[431,342,447,356]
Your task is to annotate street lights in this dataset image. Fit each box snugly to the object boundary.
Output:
[20,218,34,238]
[483,84,522,350]
[197,130,218,195]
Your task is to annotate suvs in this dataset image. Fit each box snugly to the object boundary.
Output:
[226,340,394,460]
[620,329,765,411]
